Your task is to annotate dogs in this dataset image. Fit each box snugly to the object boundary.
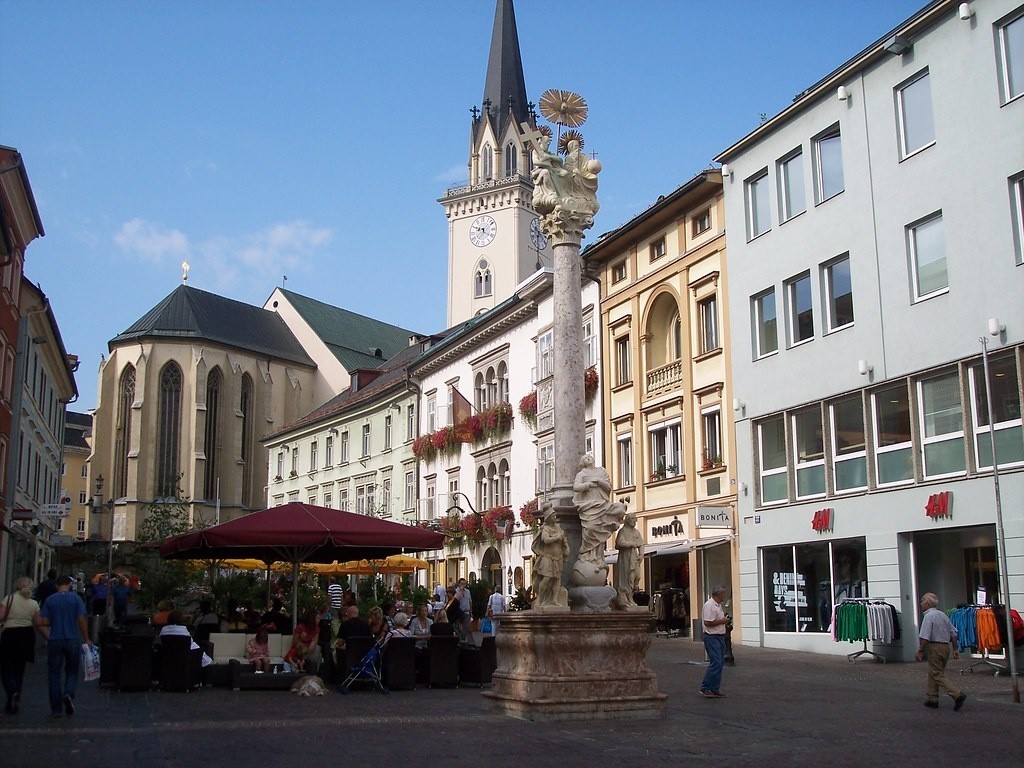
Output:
[289,675,328,697]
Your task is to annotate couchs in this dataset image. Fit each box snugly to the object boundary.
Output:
[208,633,321,689]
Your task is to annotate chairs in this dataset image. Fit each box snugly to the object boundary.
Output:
[99,628,119,683]
[120,634,154,688]
[159,634,204,688]
[418,636,460,689]
[380,637,417,692]
[461,636,497,688]
[336,637,372,675]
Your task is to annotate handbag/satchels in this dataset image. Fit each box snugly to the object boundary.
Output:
[80,643,100,681]
[488,609,493,614]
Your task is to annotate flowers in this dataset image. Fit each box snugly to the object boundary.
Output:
[463,513,481,551]
[584,371,597,403]
[482,506,514,545]
[521,499,539,534]
[414,428,456,463]
[438,515,463,551]
[520,392,537,421]
[470,404,511,442]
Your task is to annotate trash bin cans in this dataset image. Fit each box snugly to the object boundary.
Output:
[88,613,106,645]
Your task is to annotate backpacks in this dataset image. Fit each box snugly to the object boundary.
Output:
[480,615,492,633]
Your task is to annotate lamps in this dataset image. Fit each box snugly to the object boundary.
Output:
[731,394,747,413]
[722,162,736,179]
[882,35,915,59]
[856,356,876,381]
[837,84,852,101]
[957,1,976,18]
[989,317,1005,338]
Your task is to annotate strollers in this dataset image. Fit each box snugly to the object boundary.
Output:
[340,631,394,694]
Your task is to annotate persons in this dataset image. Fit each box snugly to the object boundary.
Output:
[38,569,506,682]
[37,577,93,718]
[699,587,730,698]
[572,453,628,571]
[0,576,50,714]
[918,648,924,652]
[915,593,967,711]
[535,503,570,606]
[615,513,644,608]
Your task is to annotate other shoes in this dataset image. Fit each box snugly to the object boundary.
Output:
[5,690,20,713]
[953,691,967,711]
[712,691,728,698]
[63,692,75,716]
[924,701,938,709]
[699,689,716,698]
[51,705,62,716]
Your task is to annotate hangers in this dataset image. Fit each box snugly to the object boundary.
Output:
[835,601,895,611]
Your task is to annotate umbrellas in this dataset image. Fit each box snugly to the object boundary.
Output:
[134,503,444,635]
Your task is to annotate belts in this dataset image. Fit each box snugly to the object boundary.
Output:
[928,641,949,645]
[705,632,725,637]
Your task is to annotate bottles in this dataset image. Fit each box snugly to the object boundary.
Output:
[274,665,277,674]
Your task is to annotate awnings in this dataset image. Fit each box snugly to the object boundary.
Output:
[602,535,730,566]
